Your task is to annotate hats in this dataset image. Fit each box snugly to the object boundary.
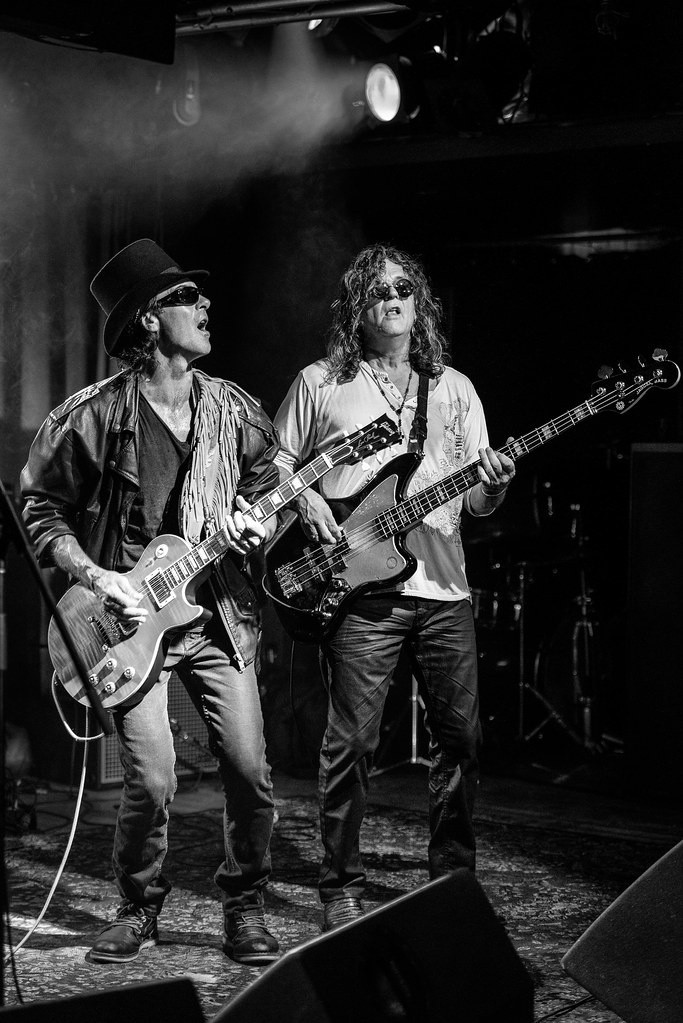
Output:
[90,238,212,357]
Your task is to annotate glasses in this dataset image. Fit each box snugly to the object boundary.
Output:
[149,286,206,315]
[373,278,412,299]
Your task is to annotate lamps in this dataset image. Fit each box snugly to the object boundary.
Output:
[363,48,440,121]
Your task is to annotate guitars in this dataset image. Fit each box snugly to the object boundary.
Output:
[259,344,682,650]
[45,408,405,714]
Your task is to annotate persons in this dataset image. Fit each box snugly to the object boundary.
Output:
[22,239,283,964]
[267,249,517,934]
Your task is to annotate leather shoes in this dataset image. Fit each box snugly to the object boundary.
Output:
[221,914,280,961]
[323,896,364,931]
[90,910,158,961]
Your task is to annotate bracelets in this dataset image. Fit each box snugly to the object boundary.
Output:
[480,483,508,497]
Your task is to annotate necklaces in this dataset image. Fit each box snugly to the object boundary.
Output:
[369,356,413,427]
